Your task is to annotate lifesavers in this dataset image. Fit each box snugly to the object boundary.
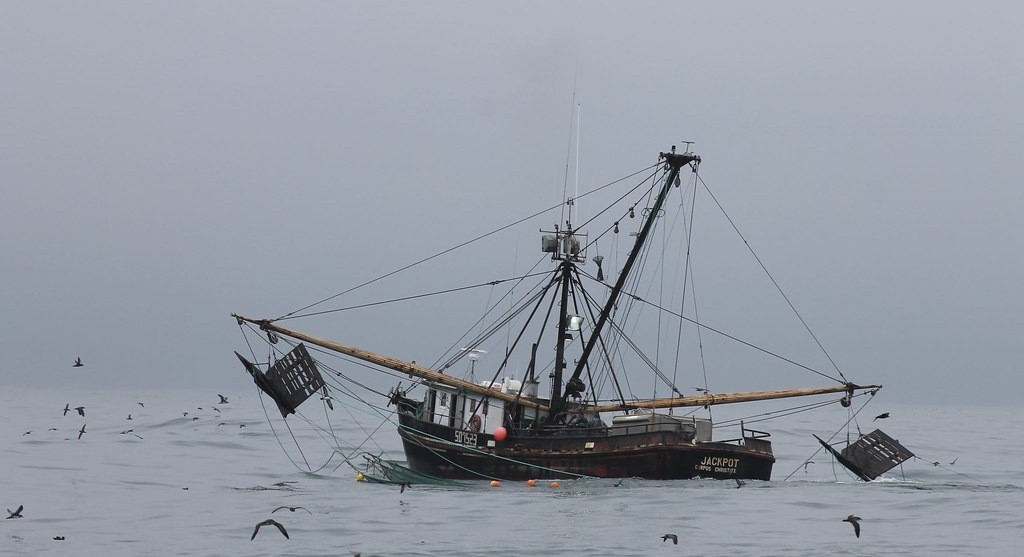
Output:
[470,414,481,432]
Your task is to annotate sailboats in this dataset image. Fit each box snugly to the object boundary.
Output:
[232,105,915,480]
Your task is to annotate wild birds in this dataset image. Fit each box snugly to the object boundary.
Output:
[6,358,890,544]
